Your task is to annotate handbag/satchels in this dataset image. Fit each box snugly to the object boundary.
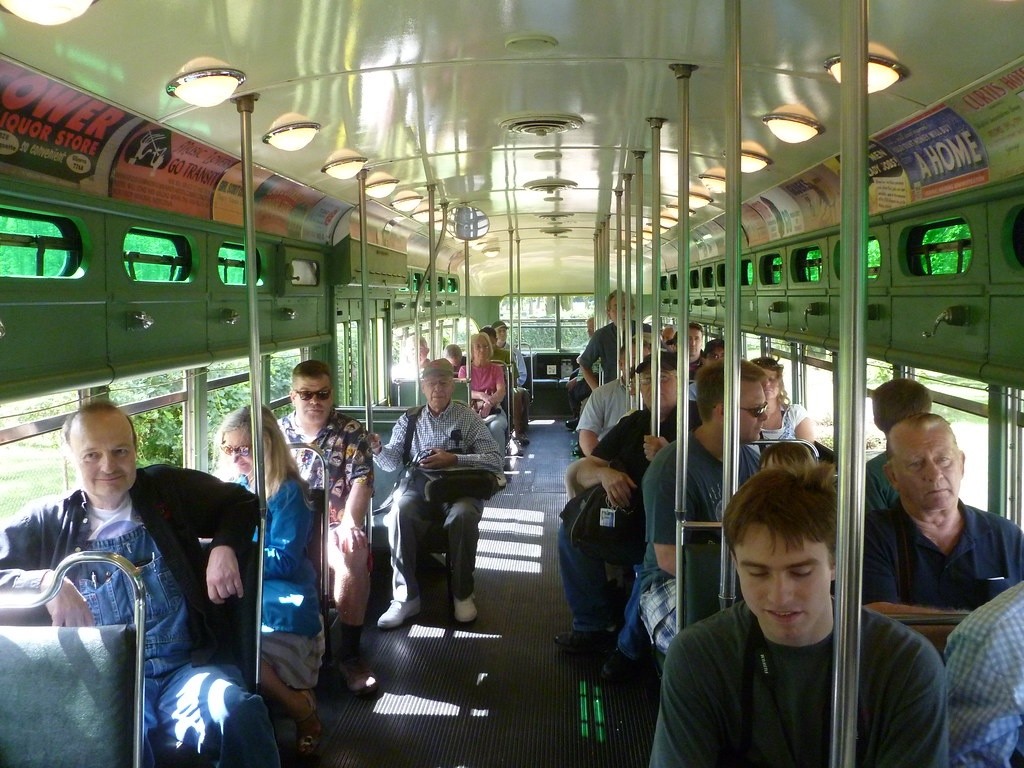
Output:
[403,459,499,504]
[469,388,503,416]
[569,457,646,568]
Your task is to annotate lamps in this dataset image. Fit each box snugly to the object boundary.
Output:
[0,0,98,26]
[631,241,636,249]
[722,150,773,175]
[410,208,439,223]
[364,179,400,199]
[432,220,488,251]
[824,53,909,96]
[165,68,247,109]
[659,216,679,228]
[321,157,368,180]
[632,236,651,245]
[389,195,423,212]
[643,230,652,240]
[688,192,714,209]
[761,113,827,144]
[698,174,727,193]
[646,223,669,234]
[262,122,321,152]
[482,248,501,257]
[665,205,697,217]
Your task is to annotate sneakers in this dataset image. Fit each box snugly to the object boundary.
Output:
[554,623,622,651]
[376,595,421,628]
[454,589,478,623]
[601,647,645,679]
[335,645,378,695]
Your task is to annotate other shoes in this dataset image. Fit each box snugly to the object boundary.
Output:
[504,438,524,456]
[566,417,579,429]
[496,474,507,486]
[573,443,584,455]
[512,430,529,446]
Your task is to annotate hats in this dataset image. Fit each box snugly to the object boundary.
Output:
[492,321,508,331]
[636,350,678,374]
[423,358,454,379]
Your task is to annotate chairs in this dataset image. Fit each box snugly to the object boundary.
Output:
[0,350,972,768]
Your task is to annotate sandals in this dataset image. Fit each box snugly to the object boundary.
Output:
[293,689,324,756]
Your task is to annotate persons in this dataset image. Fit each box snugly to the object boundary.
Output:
[480,326,522,447]
[0,402,281,768]
[214,404,325,757]
[688,337,729,400]
[648,464,948,768]
[858,412,1024,608]
[748,358,815,451]
[688,323,704,383]
[278,359,376,698]
[457,333,508,462]
[492,320,529,445]
[397,335,432,379]
[437,342,473,378]
[660,323,677,351]
[567,315,594,397]
[756,440,816,475]
[579,288,651,391]
[367,358,505,629]
[552,337,768,678]
[864,378,932,511]
[942,583,1024,768]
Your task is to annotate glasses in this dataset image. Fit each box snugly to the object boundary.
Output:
[294,386,333,401]
[706,352,724,361]
[713,400,769,418]
[221,444,252,457]
[640,375,673,386]
[472,345,489,351]
